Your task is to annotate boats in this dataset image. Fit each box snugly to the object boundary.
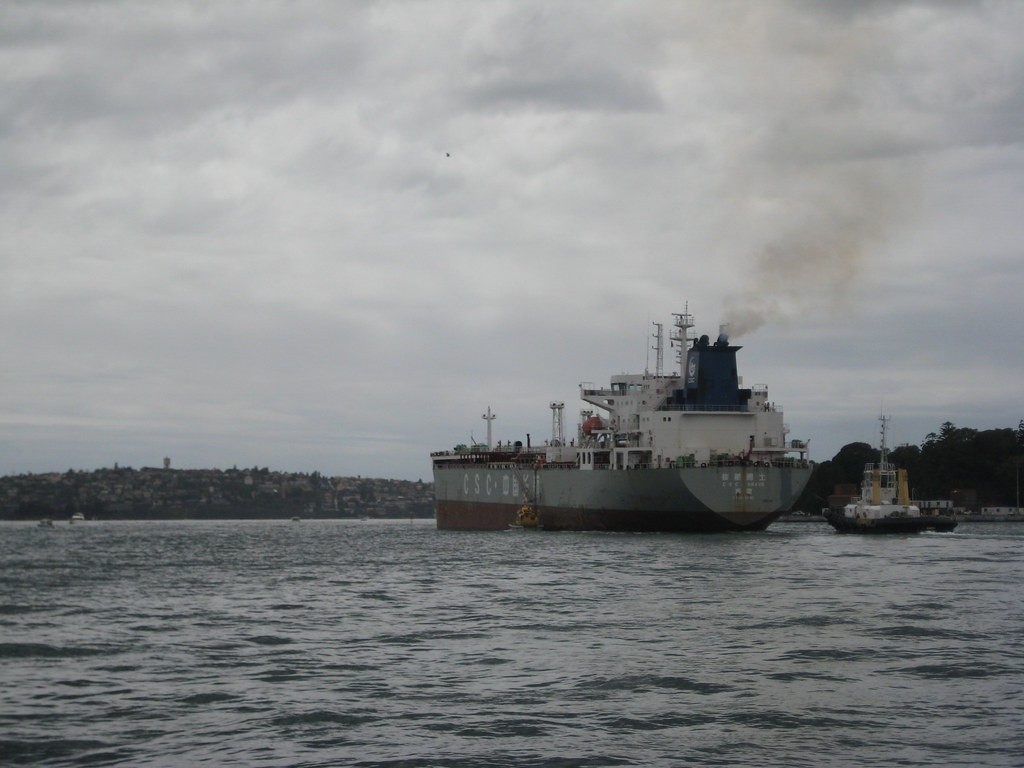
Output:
[72,512,85,520]
[429,300,817,533]
[817,411,961,537]
